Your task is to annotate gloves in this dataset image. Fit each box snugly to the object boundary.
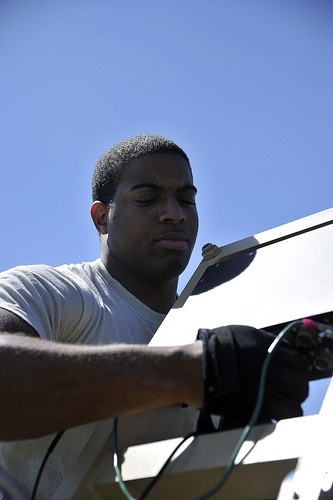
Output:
[199,325,309,430]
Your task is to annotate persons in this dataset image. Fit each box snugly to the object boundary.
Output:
[0,134,311,500]
